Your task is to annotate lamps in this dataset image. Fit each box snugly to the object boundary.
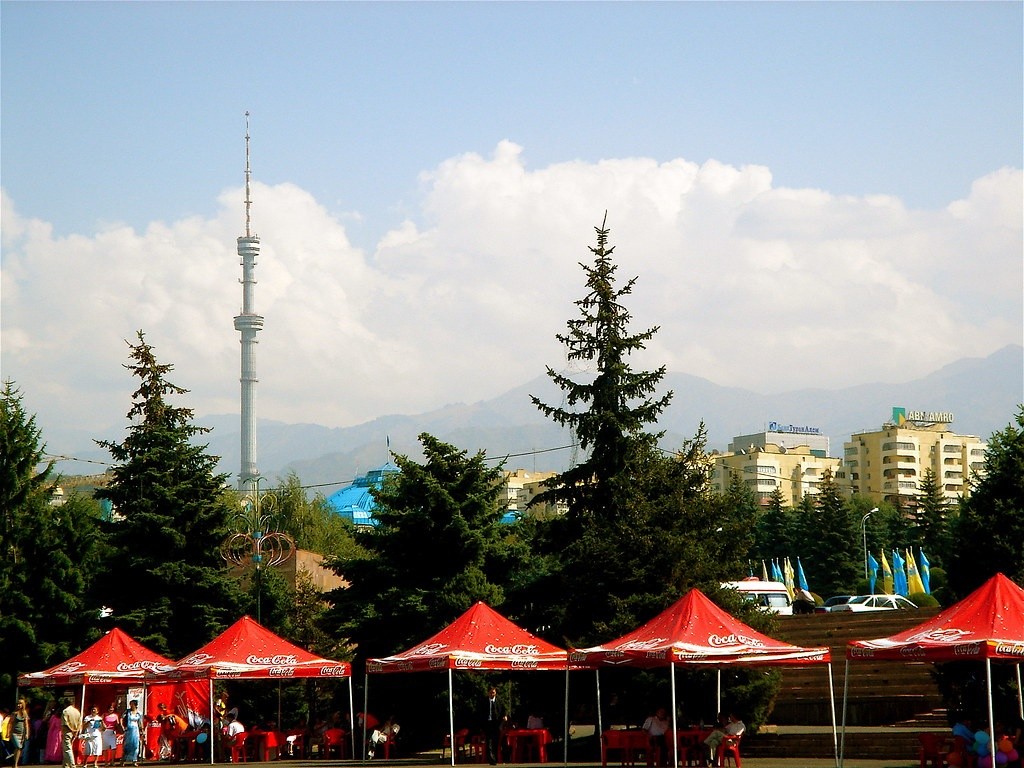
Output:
[766,443,786,454]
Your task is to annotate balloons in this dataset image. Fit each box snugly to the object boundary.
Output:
[947,752,962,765]
[974,730,1019,768]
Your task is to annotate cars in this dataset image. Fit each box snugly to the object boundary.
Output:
[814,596,858,613]
[831,594,919,612]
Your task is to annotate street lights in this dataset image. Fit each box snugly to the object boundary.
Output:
[863,506,880,577]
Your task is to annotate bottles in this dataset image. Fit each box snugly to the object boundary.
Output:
[700,720,704,730]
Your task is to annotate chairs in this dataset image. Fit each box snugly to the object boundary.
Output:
[173,724,307,762]
[384,732,397,759]
[918,731,974,768]
[716,732,744,768]
[470,728,488,764]
[323,727,347,760]
[442,728,469,764]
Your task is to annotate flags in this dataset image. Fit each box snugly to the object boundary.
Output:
[869,549,930,595]
[762,560,808,598]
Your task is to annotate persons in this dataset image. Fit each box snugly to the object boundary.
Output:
[952,716,990,754]
[592,692,623,759]
[477,685,508,766]
[792,588,815,615]
[994,719,1024,768]
[156,691,245,762]
[0,697,154,768]
[492,703,565,752]
[642,708,688,767]
[260,707,401,760]
[704,711,746,768]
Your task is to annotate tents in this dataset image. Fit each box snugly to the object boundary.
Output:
[362,601,604,766]
[840,573,1024,768]
[15,626,213,759]
[140,616,354,765]
[564,588,838,768]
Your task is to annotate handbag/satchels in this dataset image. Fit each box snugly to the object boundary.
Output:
[125,710,129,727]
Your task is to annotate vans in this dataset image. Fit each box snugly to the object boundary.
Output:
[719,576,793,616]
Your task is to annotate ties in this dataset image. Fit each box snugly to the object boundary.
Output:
[491,701,494,721]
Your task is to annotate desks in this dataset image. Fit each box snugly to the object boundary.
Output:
[663,727,713,768]
[72,733,124,764]
[505,728,554,763]
[600,729,651,767]
